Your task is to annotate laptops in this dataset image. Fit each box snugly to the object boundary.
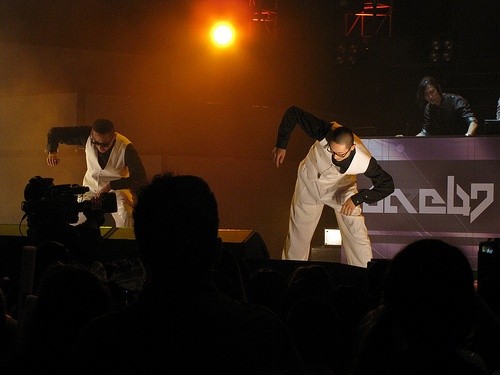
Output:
[484,120,500,136]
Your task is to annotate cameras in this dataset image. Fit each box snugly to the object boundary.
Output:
[479,242,496,254]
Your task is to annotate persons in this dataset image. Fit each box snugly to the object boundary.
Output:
[395,75,480,138]
[46,118,147,229]
[0,241,500,374]
[271,104,394,269]
[57,175,305,375]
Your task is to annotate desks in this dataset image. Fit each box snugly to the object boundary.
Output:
[355,136,500,273]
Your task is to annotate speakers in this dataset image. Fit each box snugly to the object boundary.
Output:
[216,230,272,263]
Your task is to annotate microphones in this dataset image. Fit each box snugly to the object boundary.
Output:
[69,187,89,194]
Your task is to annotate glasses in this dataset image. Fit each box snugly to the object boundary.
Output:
[327,143,353,157]
[90,132,115,148]
